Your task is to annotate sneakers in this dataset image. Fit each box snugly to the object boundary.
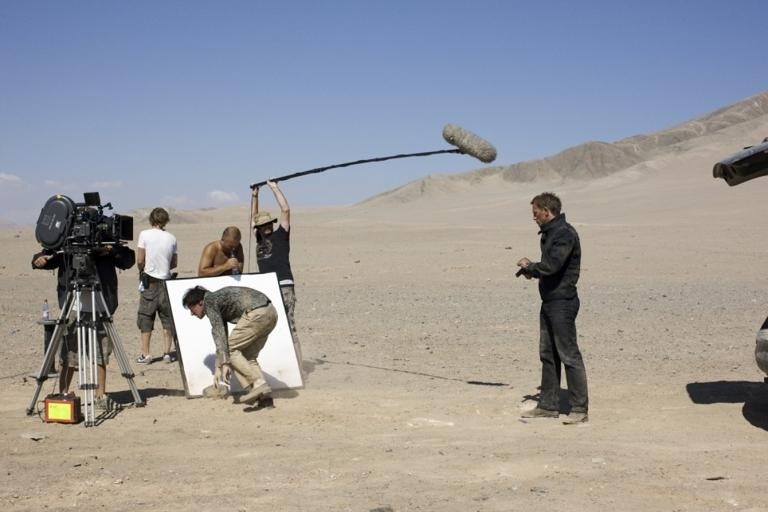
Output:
[136,355,152,364]
[520,406,560,417]
[94,393,123,412]
[562,411,588,424]
[162,353,171,362]
[239,383,273,407]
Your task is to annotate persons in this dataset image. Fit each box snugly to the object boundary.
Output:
[30,239,136,410]
[250,178,309,385]
[197,225,244,277]
[182,285,278,415]
[136,207,179,364]
[514,191,590,426]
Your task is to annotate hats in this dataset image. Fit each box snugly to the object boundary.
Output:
[252,212,277,228]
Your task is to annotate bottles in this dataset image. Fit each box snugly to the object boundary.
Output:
[42,298,49,320]
[231,250,240,274]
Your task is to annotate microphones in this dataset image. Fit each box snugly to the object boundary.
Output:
[442,124,497,163]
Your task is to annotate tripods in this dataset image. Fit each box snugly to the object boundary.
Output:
[26,277,145,427]
